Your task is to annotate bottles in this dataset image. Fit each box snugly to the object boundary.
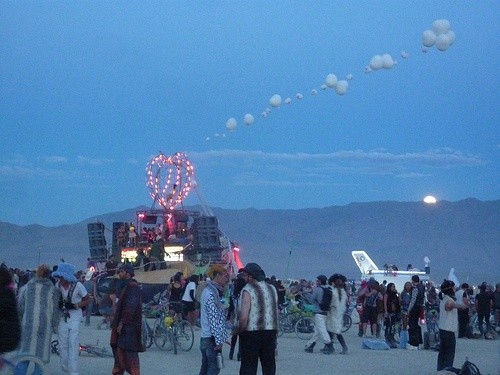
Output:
[216,350,224,368]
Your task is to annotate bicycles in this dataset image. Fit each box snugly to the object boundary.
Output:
[50,339,114,358]
[276,299,316,340]
[141,296,194,354]
[341,313,352,333]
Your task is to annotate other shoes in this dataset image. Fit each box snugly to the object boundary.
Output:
[320,345,329,350]
[340,346,348,353]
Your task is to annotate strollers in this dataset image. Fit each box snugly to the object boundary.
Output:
[97,295,117,329]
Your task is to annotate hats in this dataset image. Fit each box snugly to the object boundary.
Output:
[51,262,78,282]
[244,262,265,282]
[120,264,135,277]
[316,275,327,281]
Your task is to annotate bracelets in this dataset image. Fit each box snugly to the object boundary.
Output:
[76,304,78,308]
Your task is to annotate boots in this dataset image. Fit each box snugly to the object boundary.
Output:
[323,342,334,354]
[305,342,316,353]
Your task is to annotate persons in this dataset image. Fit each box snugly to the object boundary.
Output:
[168,272,206,326]
[266,273,348,354]
[235,263,279,375]
[0,258,95,375]
[98,290,116,328]
[116,220,169,246]
[110,264,145,375]
[105,256,117,276]
[230,268,246,361]
[351,276,500,350]
[437,279,471,375]
[200,265,228,375]
[148,240,169,270]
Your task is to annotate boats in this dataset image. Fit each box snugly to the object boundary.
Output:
[88,151,245,304]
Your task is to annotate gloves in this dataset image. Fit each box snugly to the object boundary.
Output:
[58,301,63,309]
[170,277,173,282]
[65,302,78,310]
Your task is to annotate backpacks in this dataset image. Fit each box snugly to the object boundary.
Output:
[315,285,332,310]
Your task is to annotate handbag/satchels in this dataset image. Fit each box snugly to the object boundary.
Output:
[461,361,480,374]
[365,291,378,311]
[399,330,409,348]
